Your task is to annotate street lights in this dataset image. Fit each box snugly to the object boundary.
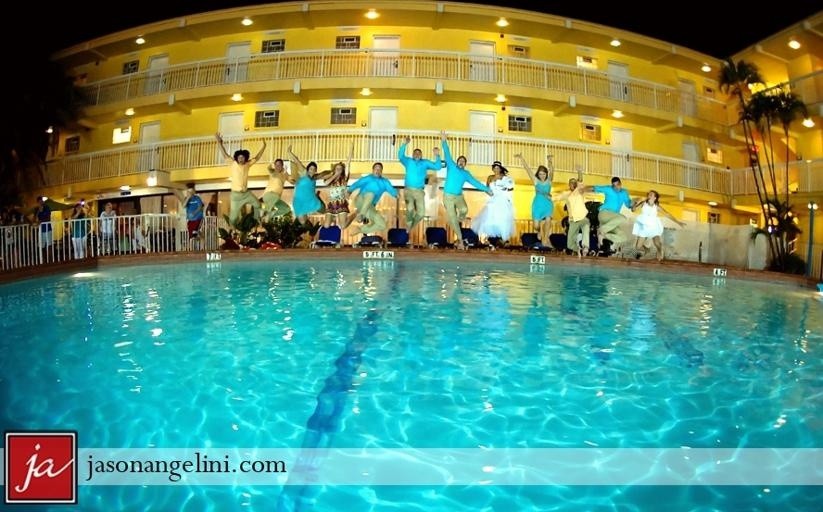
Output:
[803,200,819,279]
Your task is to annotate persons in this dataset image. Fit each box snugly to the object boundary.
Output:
[550,177,594,259]
[215,133,267,227]
[181,183,205,240]
[32,196,52,262]
[580,177,639,242]
[99,203,118,255]
[348,163,400,236]
[263,159,291,223]
[132,221,148,254]
[71,204,90,260]
[471,161,513,248]
[632,190,687,262]
[323,162,351,230]
[289,144,330,226]
[514,153,554,248]
[398,135,441,232]
[440,129,492,250]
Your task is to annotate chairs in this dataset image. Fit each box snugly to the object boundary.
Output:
[386,227,410,248]
[424,227,455,249]
[488,236,518,252]
[314,225,341,248]
[519,233,552,253]
[351,236,384,249]
[460,227,494,250]
[548,233,573,255]
